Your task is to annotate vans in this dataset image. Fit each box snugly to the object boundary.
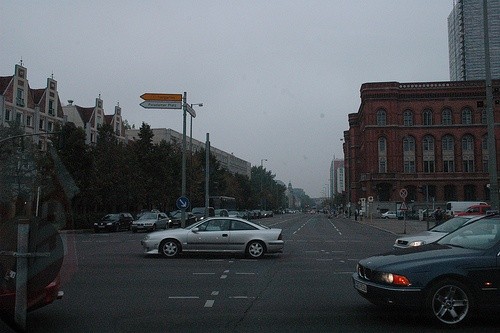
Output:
[446,202,493,218]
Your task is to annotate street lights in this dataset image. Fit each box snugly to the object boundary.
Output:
[261,158,268,210]
[187,103,203,211]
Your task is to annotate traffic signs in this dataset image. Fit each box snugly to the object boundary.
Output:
[140,93,196,117]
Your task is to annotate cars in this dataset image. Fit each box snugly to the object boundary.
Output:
[168,207,273,227]
[93,213,133,231]
[382,211,398,220]
[131,211,171,231]
[0,253,65,320]
[140,217,284,259]
[273,209,335,215]
[352,214,500,328]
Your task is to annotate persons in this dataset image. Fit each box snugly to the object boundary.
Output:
[434,207,446,225]
